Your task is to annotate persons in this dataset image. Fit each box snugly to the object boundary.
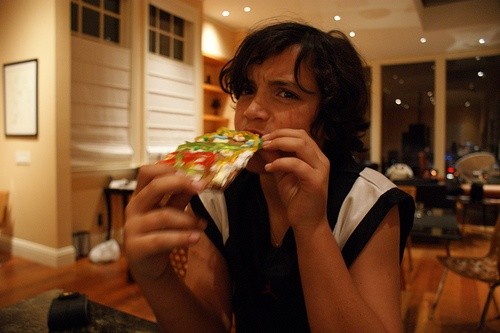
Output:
[122,22,415,333]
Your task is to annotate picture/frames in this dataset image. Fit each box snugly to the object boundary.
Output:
[4,58,38,137]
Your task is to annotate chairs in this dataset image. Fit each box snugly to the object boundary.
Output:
[430,203,500,327]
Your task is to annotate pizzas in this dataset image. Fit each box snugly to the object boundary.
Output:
[151,128,263,192]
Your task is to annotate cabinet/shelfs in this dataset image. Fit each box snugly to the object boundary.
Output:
[202,55,231,132]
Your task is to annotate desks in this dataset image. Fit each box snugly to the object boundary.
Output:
[0,288,163,332]
